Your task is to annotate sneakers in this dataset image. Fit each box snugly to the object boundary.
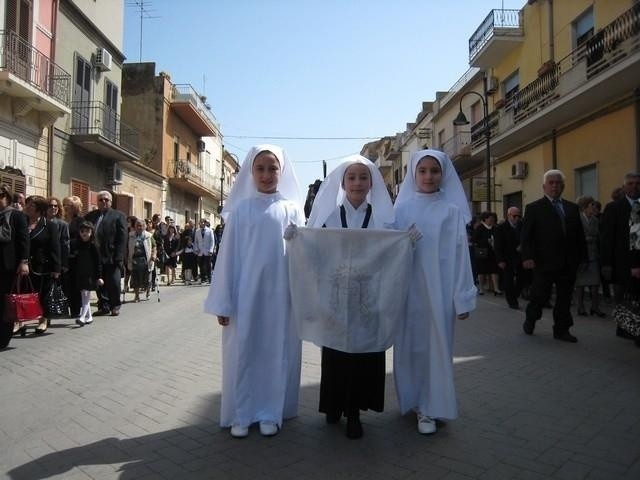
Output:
[326,412,342,422]
[346,416,363,439]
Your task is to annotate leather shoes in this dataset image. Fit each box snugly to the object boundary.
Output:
[578,307,587,316]
[75,319,85,327]
[259,420,278,435]
[110,305,120,316]
[553,328,578,343]
[415,412,437,434]
[231,424,248,438]
[86,316,93,324]
[92,309,111,317]
[523,318,536,335]
[590,309,607,317]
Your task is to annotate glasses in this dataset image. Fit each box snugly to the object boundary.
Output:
[0,192,8,199]
[98,198,109,202]
[49,204,58,208]
[510,213,522,219]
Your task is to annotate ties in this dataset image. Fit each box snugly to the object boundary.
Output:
[554,201,566,233]
[94,213,104,234]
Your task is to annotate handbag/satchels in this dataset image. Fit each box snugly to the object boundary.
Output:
[3,269,43,323]
[45,272,69,319]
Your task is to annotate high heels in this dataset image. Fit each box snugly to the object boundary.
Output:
[35,324,47,335]
[494,290,503,296]
[11,324,27,338]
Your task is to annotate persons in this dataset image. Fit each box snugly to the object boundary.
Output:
[202,143,306,437]
[282,154,424,440]
[493,206,528,309]
[0,182,30,350]
[128,217,157,303]
[84,189,129,317]
[193,218,215,285]
[44,196,71,281]
[520,169,591,344]
[72,220,105,328]
[11,197,61,335]
[390,149,479,435]
[472,211,505,297]
[119,216,139,293]
[153,212,196,286]
[60,196,85,318]
[600,170,640,346]
[144,217,160,292]
[575,192,608,318]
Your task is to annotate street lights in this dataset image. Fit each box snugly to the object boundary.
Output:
[451,89,492,212]
[219,145,239,223]
